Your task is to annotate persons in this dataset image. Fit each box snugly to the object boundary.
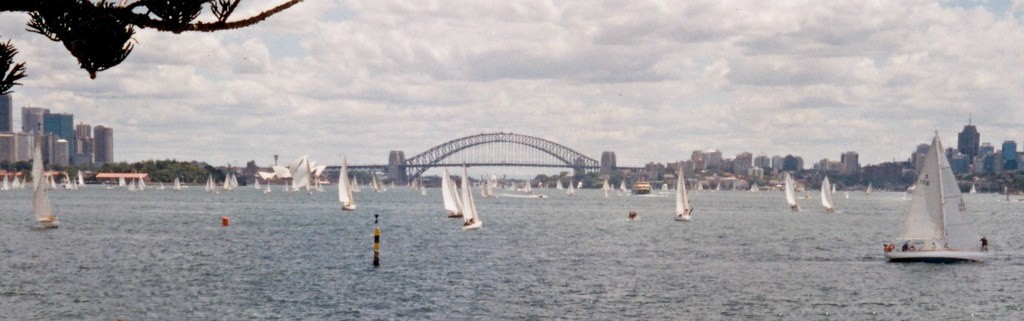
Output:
[884,240,916,252]
[980,237,988,251]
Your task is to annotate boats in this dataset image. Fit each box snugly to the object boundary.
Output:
[631,175,652,195]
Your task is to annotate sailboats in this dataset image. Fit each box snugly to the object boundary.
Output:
[782,171,839,214]
[864,180,876,196]
[172,178,185,191]
[291,172,326,193]
[481,174,585,198]
[203,171,240,193]
[881,129,989,263]
[0,169,87,192]
[675,166,693,220]
[601,176,628,195]
[691,180,724,193]
[254,179,272,193]
[338,162,386,211]
[26,168,60,230]
[442,160,483,230]
[119,170,149,194]
